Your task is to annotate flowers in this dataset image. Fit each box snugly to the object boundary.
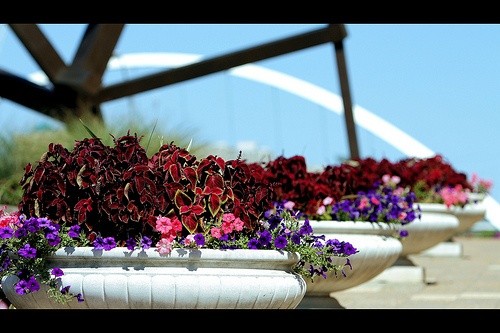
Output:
[278,154,422,240]
[0,115,359,304]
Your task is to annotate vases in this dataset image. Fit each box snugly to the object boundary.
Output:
[0,246,308,308]
[284,219,405,298]
[394,153,493,266]
